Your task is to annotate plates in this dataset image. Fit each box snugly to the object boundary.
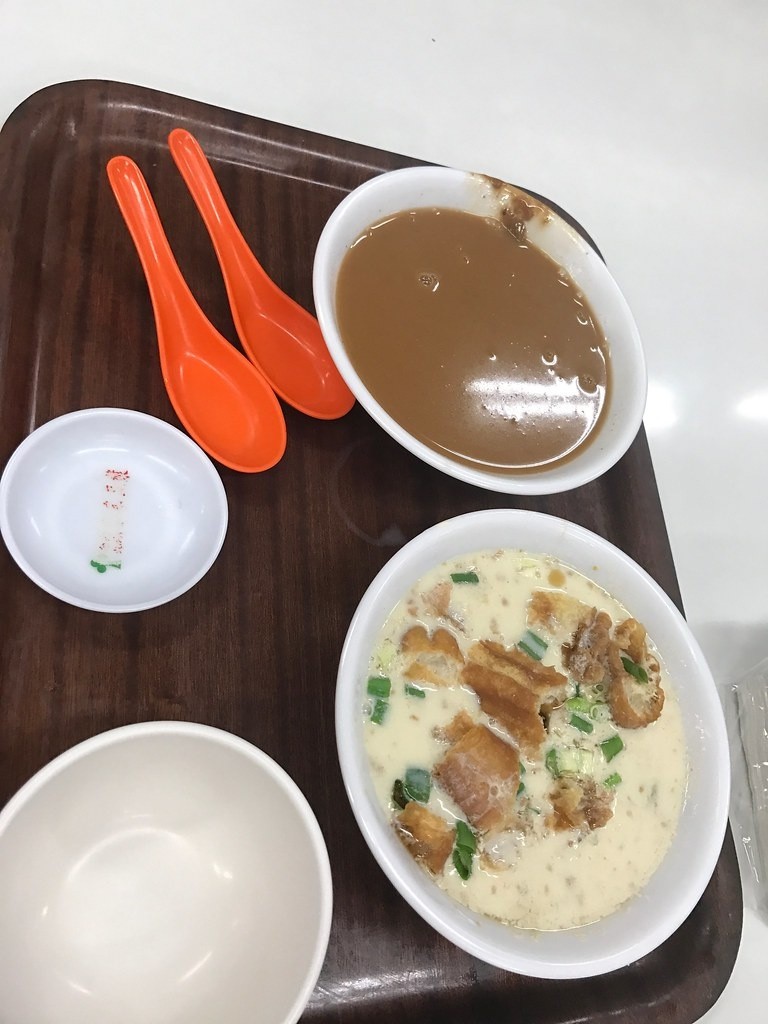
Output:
[0,408,231,615]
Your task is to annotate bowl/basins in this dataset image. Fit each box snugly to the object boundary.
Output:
[315,167,646,497]
[1,718,333,1024]
[333,506,730,979]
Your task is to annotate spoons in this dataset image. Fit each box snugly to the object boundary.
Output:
[169,128,356,422]
[109,156,287,474]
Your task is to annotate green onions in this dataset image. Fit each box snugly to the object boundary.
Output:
[365,567,649,878]
